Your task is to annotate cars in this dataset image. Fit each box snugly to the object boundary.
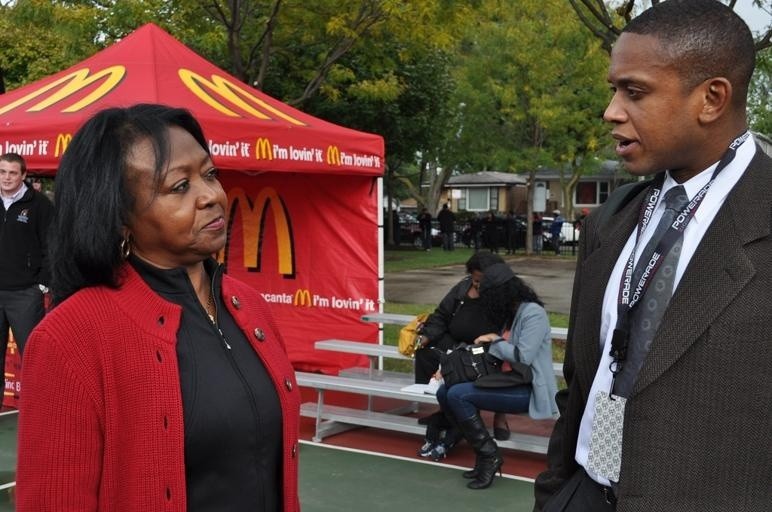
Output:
[396,210,579,251]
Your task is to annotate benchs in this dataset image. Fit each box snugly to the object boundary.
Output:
[293,312,569,456]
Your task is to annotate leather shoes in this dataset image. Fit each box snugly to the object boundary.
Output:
[494,414,510,440]
[419,416,431,425]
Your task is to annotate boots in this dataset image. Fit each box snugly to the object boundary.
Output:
[446,413,503,489]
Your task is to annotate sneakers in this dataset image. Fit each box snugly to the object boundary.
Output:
[420,438,449,461]
[424,377,445,395]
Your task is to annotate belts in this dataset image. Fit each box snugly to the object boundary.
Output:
[578,465,616,505]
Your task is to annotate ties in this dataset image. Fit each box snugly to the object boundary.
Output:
[613,185,689,399]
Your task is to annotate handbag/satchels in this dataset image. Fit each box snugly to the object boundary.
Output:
[473,362,533,388]
[398,312,430,358]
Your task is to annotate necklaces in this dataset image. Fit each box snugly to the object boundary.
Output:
[201,282,216,323]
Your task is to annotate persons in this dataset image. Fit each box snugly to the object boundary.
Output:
[436,202,457,252]
[14,100,303,510]
[462,207,589,256]
[436,259,560,489]
[2,151,55,406]
[532,0,771,511]
[416,207,432,251]
[407,252,505,460]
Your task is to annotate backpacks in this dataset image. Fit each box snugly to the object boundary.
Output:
[429,342,503,388]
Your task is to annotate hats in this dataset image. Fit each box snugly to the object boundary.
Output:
[552,209,560,215]
[478,263,514,293]
